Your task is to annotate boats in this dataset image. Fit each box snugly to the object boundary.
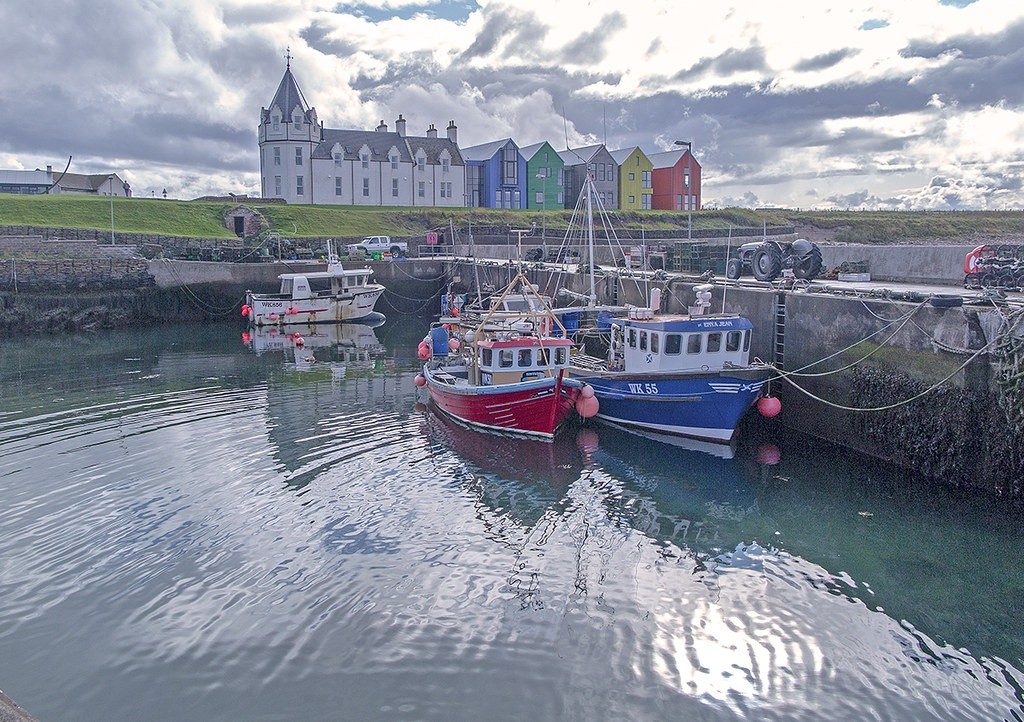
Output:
[244,237,386,326]
[421,167,773,458]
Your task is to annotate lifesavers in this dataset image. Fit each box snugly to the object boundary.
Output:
[541,317,553,336]
[446,325,451,330]
[969,251,994,272]
[929,297,964,307]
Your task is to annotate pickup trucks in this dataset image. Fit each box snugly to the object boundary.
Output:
[342,235,408,259]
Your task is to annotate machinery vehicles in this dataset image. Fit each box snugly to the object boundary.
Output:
[726,236,822,282]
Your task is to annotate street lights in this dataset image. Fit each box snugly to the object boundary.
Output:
[535,174,548,258]
[675,141,693,242]
[108,176,114,245]
